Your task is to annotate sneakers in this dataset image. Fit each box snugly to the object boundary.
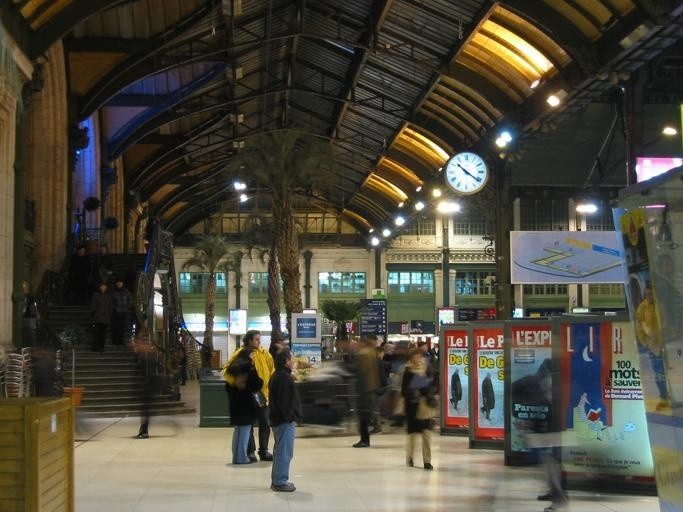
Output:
[406,457,433,469]
[352,441,369,447]
[536,492,568,511]
[449,398,458,409]
[269,481,296,491]
[132,431,149,438]
[369,426,382,433]
[233,453,273,463]
[480,406,489,420]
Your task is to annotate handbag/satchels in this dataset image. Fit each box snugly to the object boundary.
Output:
[414,395,436,420]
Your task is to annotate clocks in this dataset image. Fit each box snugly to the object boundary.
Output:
[442,150,491,197]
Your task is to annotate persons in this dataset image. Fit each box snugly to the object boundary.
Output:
[90,279,113,353]
[171,342,186,384]
[203,340,212,368]
[111,277,134,345]
[222,327,439,492]
[20,279,39,349]
[448,367,462,412]
[92,240,122,287]
[512,357,552,429]
[70,243,91,305]
[479,372,495,422]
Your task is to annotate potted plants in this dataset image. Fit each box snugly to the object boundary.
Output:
[59,328,84,406]
